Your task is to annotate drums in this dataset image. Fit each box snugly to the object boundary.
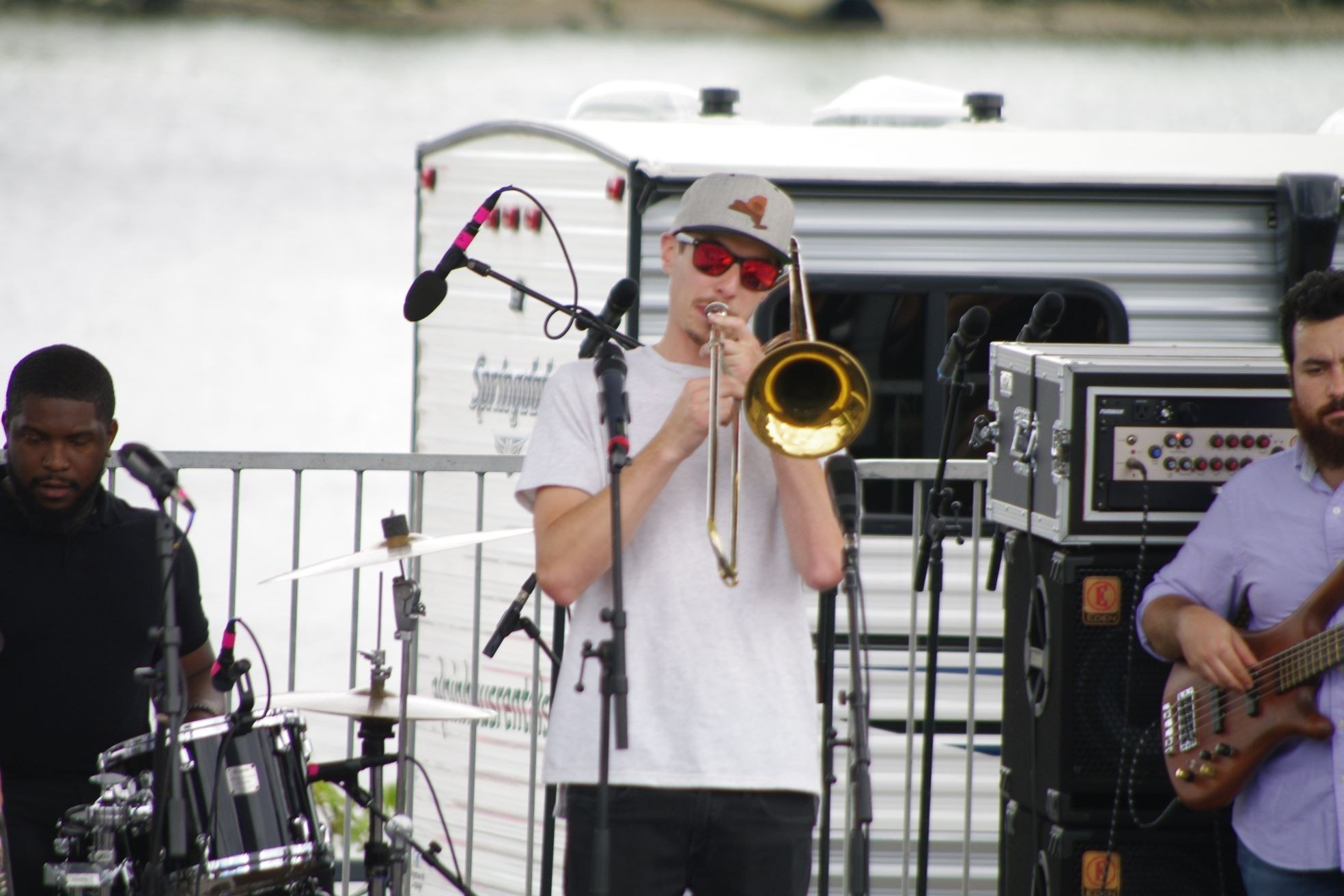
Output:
[89,699,323,896]
[41,795,153,896]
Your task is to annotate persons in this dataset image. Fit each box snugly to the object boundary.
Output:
[0,345,228,896]
[1137,271,1344,896]
[514,173,843,896]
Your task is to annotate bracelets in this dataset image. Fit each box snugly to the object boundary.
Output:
[186,704,219,716]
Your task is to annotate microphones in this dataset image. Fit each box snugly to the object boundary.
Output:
[403,191,501,323]
[482,572,537,658]
[592,343,629,467]
[936,307,990,384]
[119,444,194,513]
[577,278,640,359]
[824,456,863,557]
[210,621,236,692]
[306,754,399,783]
[1019,291,1064,343]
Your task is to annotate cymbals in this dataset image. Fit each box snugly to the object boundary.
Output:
[255,528,533,591]
[259,691,500,725]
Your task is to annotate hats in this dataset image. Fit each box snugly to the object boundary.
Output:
[666,173,796,265]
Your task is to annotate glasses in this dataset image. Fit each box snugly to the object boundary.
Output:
[677,232,782,292]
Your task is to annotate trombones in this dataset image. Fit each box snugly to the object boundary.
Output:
[701,219,878,586]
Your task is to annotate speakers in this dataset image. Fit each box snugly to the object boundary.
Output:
[1002,532,1235,829]
[998,794,1249,896]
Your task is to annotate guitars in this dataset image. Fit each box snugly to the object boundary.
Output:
[1160,555,1344,812]
